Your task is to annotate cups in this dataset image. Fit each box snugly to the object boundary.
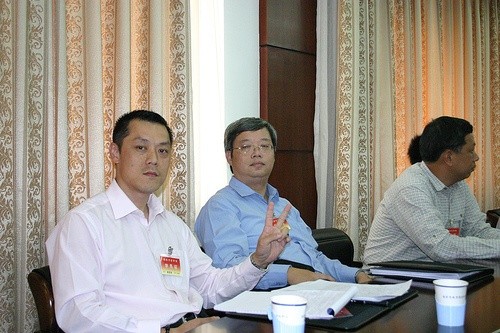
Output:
[433,279,469,327]
[270,295,307,333]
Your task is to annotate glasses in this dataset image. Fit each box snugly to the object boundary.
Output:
[232,144,276,155]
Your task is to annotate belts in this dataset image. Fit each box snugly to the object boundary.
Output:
[169,311,205,329]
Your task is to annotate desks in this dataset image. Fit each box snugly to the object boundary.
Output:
[184,259,500,333]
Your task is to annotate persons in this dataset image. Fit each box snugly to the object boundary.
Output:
[364,117,500,270]
[195,117,373,290]
[45,110,290,333]
